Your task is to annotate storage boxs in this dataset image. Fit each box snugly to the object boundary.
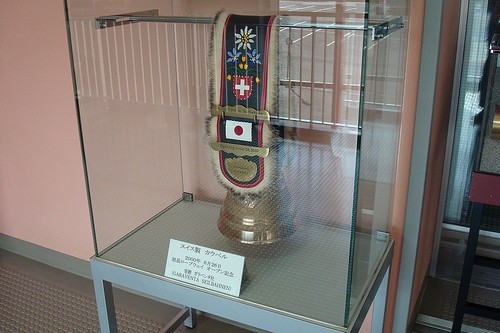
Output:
[59,0,426,333]
[451,0,500,333]
